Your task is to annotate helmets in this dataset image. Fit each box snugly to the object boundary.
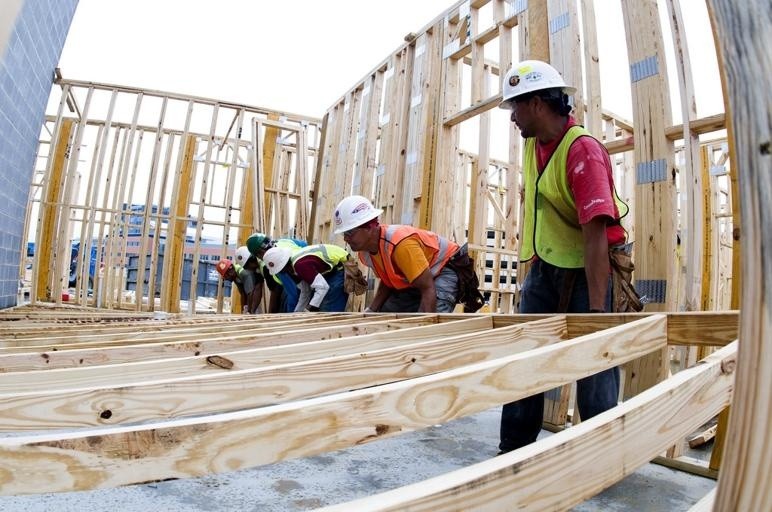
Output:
[332,195,384,235]
[216,258,233,277]
[234,246,251,268]
[499,58,577,109]
[246,232,266,255]
[263,247,291,275]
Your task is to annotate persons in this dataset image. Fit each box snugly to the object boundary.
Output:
[333,195,464,313]
[498,60,629,453]
[216,233,352,314]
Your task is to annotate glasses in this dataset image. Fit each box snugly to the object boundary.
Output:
[341,229,355,236]
[507,102,521,113]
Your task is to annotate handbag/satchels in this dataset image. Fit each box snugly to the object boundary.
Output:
[445,246,485,313]
[343,256,368,296]
[607,245,636,313]
